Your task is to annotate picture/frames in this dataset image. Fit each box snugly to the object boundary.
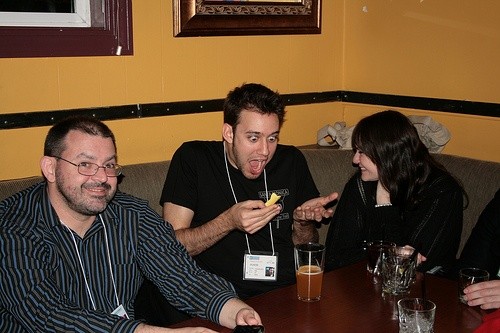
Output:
[172,0,322,38]
[0,0,134,57]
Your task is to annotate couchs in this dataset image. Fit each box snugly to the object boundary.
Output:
[0,148,500,261]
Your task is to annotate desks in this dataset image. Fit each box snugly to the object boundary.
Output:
[168,259,497,333]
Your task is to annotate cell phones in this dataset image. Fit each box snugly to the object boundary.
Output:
[233,325,264,333]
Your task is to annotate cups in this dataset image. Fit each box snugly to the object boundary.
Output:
[362,238,396,275]
[398,298,436,333]
[293,242,326,303]
[381,247,417,295]
[457,267,490,305]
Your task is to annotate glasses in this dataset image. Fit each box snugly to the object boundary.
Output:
[52,154,124,177]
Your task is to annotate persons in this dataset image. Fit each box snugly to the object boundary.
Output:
[323,110,470,275]
[0,113,267,333]
[159,82,339,301]
[445,190,500,310]
